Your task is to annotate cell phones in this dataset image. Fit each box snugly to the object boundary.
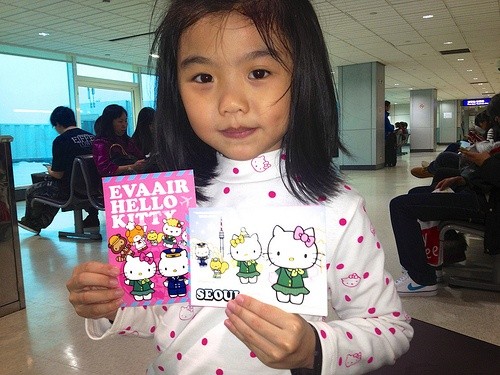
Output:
[43,164,51,168]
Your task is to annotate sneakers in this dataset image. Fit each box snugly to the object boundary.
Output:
[81,215,100,231]
[395,274,439,296]
[449,247,466,262]
[18,221,41,233]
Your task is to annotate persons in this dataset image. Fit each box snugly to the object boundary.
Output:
[94,104,155,208]
[390,93,500,297]
[385,100,394,167]
[65,0,414,375]
[18,106,96,234]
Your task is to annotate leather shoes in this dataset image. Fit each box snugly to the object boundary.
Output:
[411,167,433,178]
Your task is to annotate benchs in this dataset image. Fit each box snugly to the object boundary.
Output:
[31,157,106,241]
[397,134,411,156]
[437,219,500,292]
